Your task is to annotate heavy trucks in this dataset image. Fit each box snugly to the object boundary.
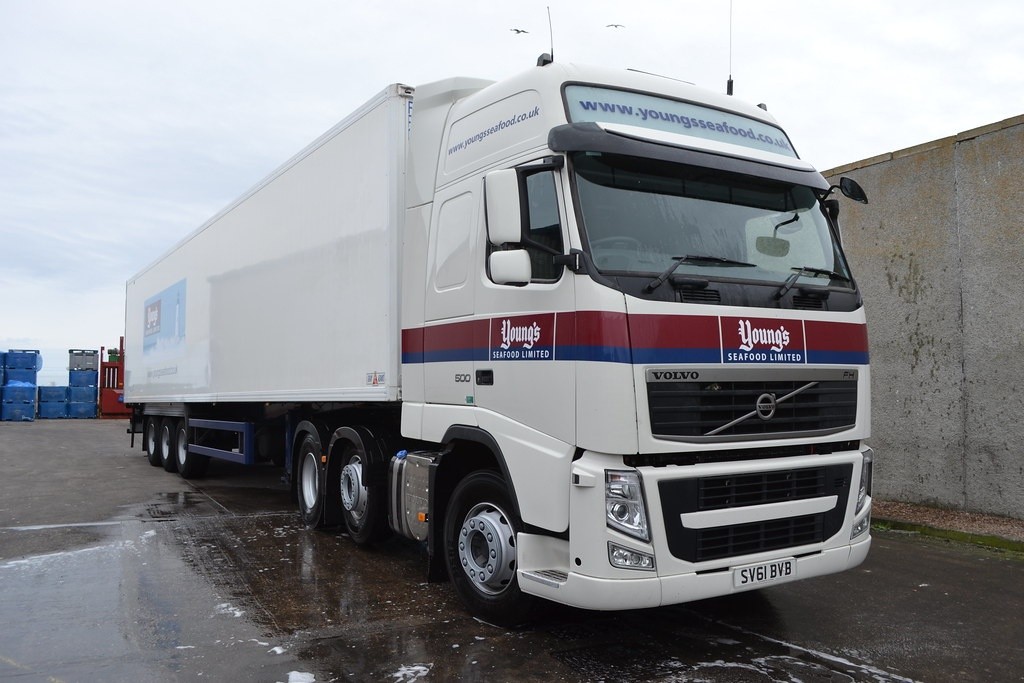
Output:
[123,54,875,618]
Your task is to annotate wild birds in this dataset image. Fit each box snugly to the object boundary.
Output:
[511,29,529,34]
[606,24,627,28]
